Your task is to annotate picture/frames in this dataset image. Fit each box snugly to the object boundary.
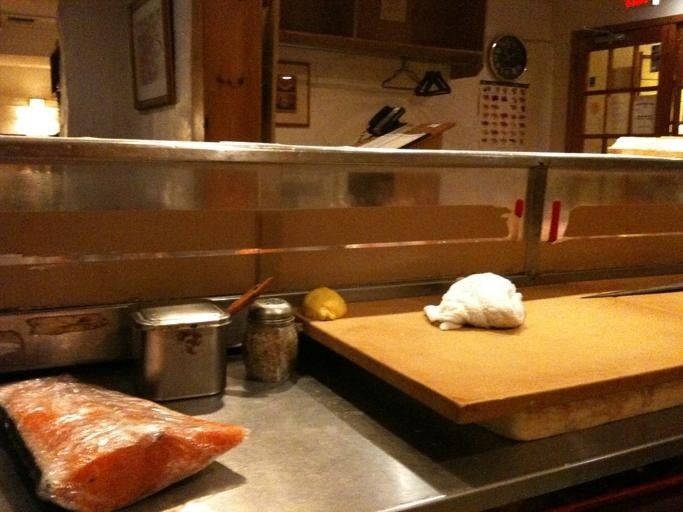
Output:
[275,60,311,128]
[128,0,176,110]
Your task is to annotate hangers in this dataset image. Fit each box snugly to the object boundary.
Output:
[414,58,451,96]
[382,57,420,90]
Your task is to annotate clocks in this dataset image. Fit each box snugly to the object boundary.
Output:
[489,33,529,81]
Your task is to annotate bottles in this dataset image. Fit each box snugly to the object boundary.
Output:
[244,297,298,384]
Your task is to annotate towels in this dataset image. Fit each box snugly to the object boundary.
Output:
[424,272,524,330]
